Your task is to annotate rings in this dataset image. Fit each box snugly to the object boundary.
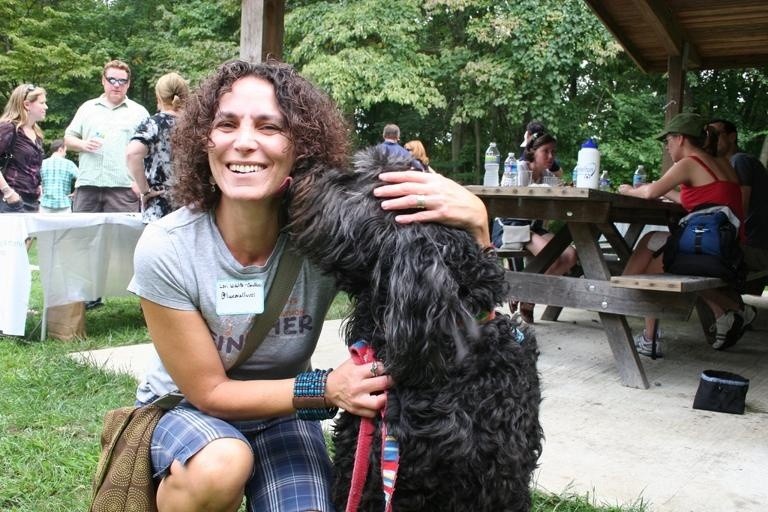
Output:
[127,57,490,512]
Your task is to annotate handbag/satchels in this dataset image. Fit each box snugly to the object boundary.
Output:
[0,120,16,175]
[90,237,304,512]
[496,218,537,252]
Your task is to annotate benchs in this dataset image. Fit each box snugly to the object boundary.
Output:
[492,247,615,323]
[609,265,768,322]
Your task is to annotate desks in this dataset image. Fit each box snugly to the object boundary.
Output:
[0,213,148,347]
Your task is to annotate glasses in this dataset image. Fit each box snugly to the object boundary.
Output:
[23,84,37,101]
[526,131,545,151]
[406,149,412,151]
[662,135,680,144]
[103,73,129,85]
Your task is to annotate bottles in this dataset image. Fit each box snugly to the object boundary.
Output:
[634,164,645,190]
[504,153,517,187]
[576,139,599,189]
[599,169,611,192]
[484,142,501,186]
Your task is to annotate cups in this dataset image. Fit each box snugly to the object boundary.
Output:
[518,170,533,187]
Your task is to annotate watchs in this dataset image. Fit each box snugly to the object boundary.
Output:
[141,189,152,198]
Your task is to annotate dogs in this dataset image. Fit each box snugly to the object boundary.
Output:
[279,141,546,512]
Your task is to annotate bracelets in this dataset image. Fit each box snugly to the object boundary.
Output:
[292,370,339,420]
[1,185,10,193]
[417,194,425,208]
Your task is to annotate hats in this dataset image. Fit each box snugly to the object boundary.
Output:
[655,113,708,141]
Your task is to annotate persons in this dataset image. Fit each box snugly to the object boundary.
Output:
[617,114,745,359]
[492,133,578,324]
[3,190,14,201]
[38,139,79,213]
[517,121,563,226]
[64,60,151,310]
[0,84,48,213]
[403,139,429,172]
[126,72,190,226]
[376,123,414,164]
[703,119,768,349]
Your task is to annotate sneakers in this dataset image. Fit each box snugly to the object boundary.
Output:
[520,302,535,323]
[736,304,756,342]
[709,311,743,349]
[509,300,520,313]
[632,330,657,358]
